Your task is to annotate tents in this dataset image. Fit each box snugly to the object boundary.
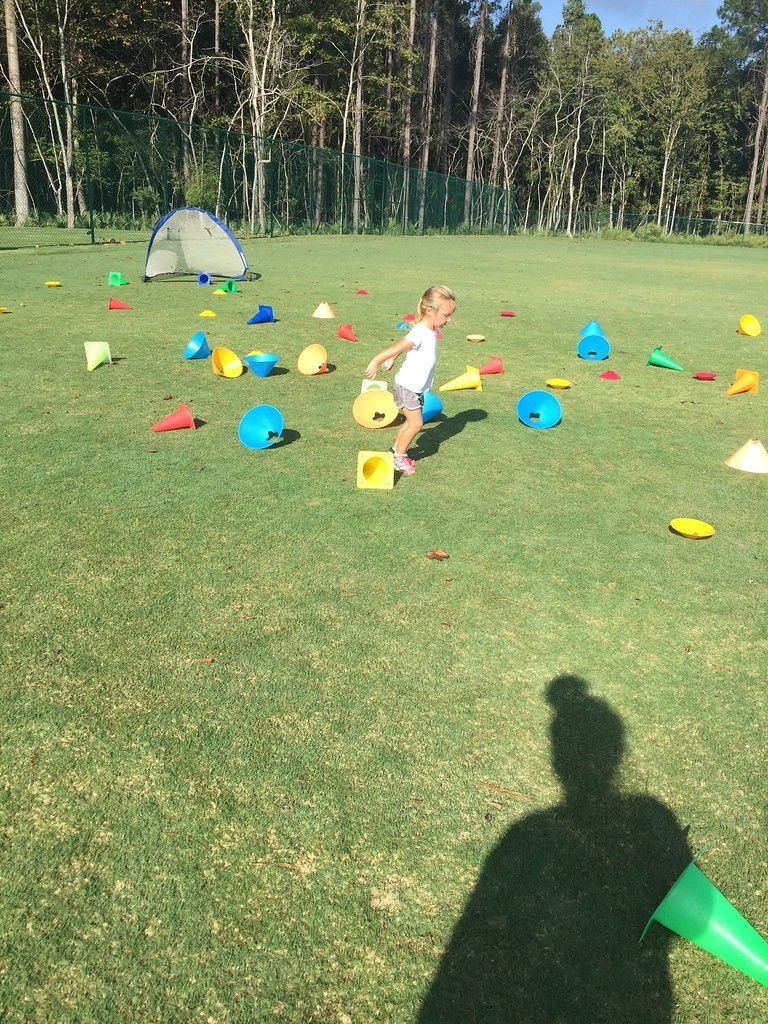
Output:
[141,206,252,283]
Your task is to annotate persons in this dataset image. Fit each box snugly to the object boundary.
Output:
[364,285,456,475]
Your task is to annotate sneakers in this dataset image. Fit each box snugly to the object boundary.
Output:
[390,448,415,467]
[394,457,415,474]
